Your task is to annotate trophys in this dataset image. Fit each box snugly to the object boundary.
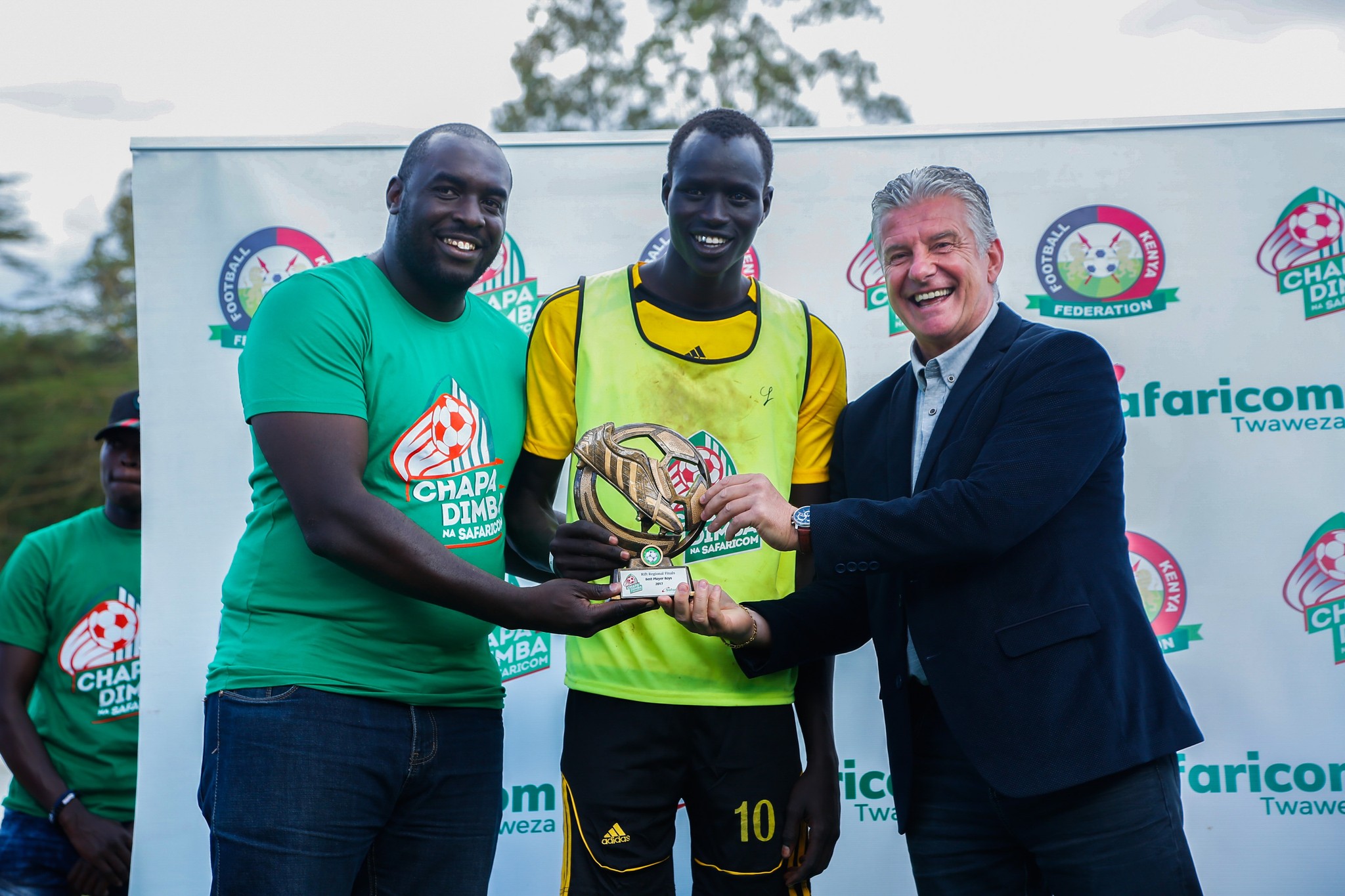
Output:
[574,422,712,605]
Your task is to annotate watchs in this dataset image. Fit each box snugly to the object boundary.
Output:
[790,505,814,551]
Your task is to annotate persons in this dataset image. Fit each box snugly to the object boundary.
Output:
[504,105,847,896]
[656,163,1212,896]
[196,123,662,896]
[1,388,141,896]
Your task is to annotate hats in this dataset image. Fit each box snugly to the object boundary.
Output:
[95,390,140,440]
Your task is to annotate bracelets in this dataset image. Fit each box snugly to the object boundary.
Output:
[720,603,758,648]
[52,789,76,822]
